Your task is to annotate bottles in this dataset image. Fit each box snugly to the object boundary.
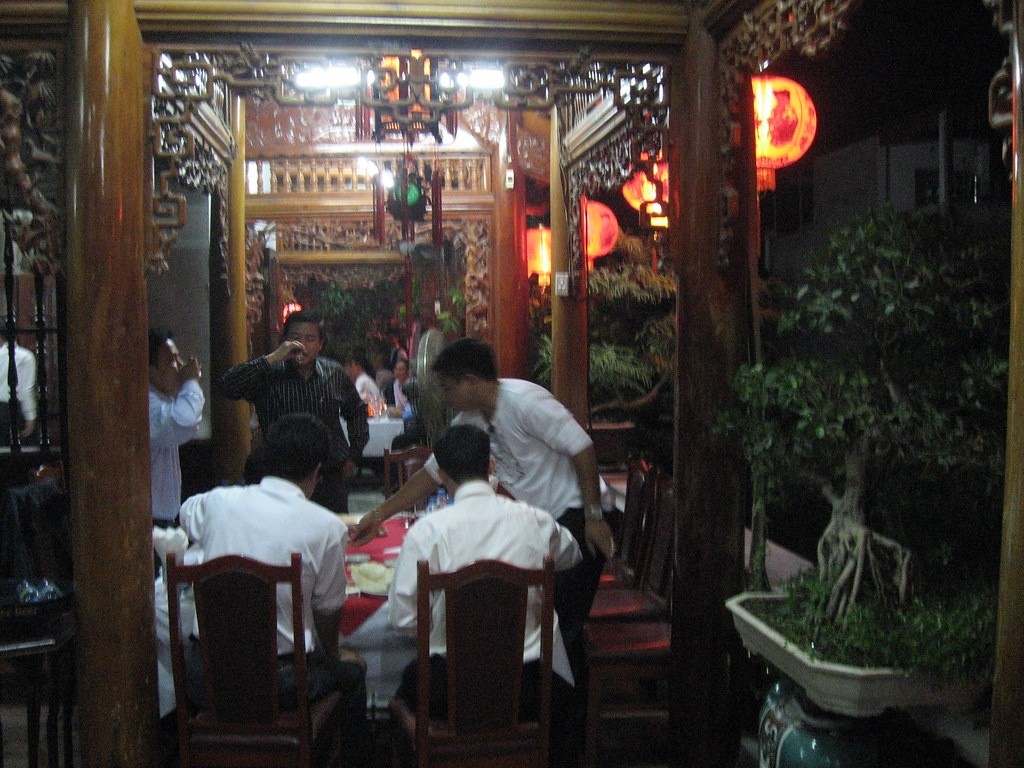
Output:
[38,576,58,602]
[425,497,439,514]
[17,579,39,604]
[437,488,446,508]
[447,494,453,506]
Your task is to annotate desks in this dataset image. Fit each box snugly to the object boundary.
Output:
[0,600,80,768]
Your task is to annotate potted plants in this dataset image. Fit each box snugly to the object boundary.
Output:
[531,334,659,472]
[714,189,1009,716]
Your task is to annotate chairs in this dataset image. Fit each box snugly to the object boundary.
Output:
[165,549,343,768]
[388,556,556,767]
[384,444,435,512]
[581,456,678,768]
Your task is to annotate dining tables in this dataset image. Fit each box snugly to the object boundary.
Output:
[156,512,575,718]
[337,416,404,459]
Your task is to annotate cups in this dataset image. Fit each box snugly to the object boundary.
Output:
[295,350,306,363]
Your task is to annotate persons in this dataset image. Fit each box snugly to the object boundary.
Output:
[220,309,370,513]
[388,426,581,727]
[348,356,385,418]
[385,359,420,451]
[388,331,408,365]
[149,327,205,580]
[0,331,37,448]
[352,336,614,768]
[180,412,372,768]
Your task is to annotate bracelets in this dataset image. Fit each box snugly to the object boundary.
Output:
[584,505,603,520]
[371,507,383,522]
